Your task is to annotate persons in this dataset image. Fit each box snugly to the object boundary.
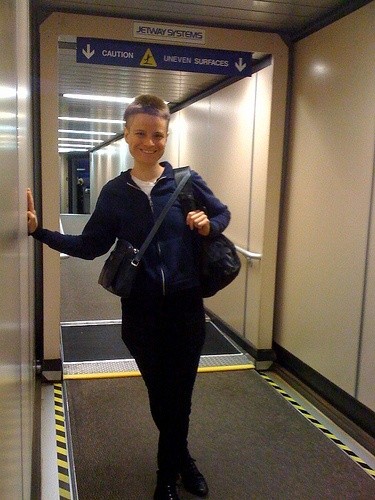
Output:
[75,178,85,214]
[28,92,234,500]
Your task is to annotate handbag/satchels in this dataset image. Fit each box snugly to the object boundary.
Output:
[99,243,141,299]
[174,166,242,300]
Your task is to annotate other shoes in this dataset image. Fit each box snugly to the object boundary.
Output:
[177,453,210,496]
[154,481,177,500]
